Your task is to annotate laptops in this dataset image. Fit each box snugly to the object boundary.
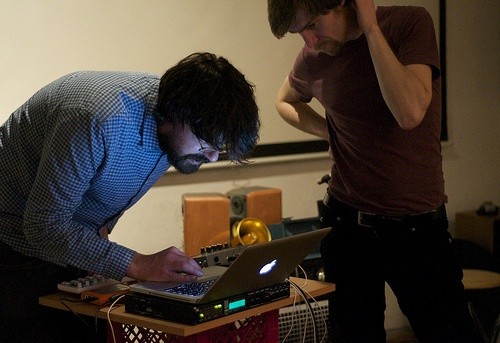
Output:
[129,227,332,303]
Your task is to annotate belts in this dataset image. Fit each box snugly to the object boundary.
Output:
[323,193,445,230]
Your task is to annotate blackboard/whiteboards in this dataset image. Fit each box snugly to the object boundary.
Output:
[0,0,448,172]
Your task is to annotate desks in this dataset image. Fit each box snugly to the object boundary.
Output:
[37,265,337,342]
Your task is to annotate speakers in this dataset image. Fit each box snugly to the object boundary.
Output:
[181,193,230,258]
[227,186,281,225]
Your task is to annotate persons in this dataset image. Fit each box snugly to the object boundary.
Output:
[0,52,263,343]
[268,0,475,343]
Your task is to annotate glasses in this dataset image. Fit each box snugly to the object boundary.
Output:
[196,135,229,154]
